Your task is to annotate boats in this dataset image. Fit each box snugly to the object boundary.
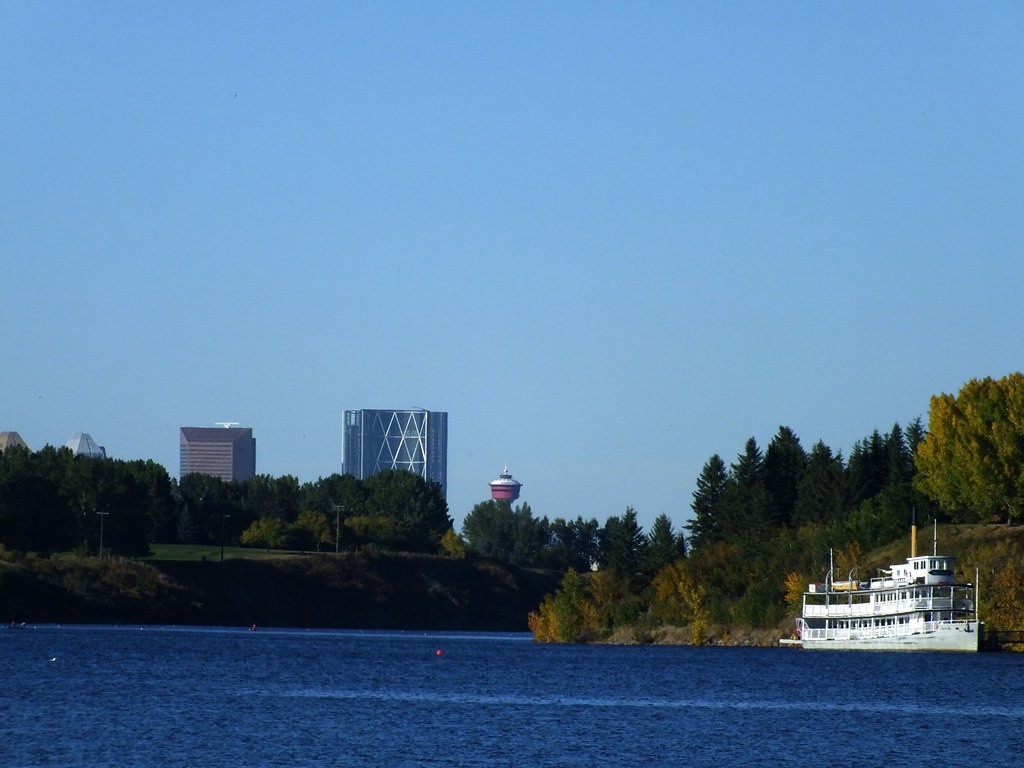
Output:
[776,505,980,653]
[832,580,860,591]
